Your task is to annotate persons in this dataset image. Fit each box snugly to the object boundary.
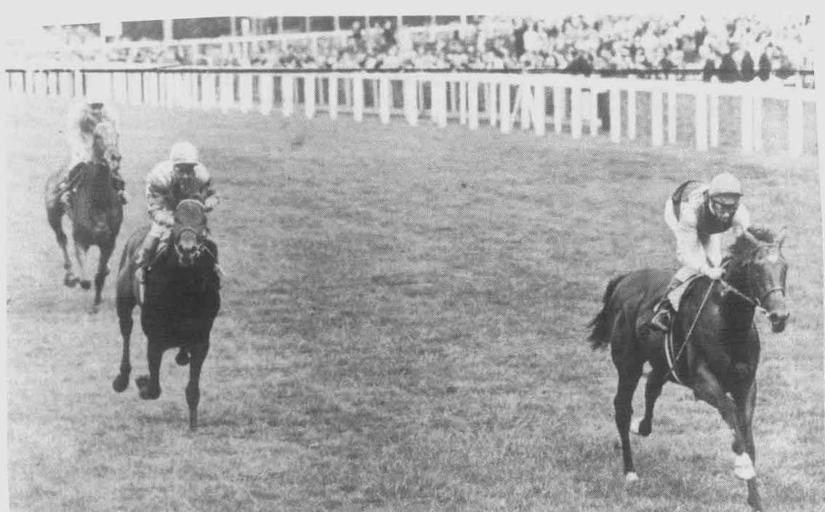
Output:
[653,173,750,333]
[57,98,127,209]
[133,142,222,283]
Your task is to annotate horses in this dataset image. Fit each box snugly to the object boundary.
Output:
[582,226,790,512]
[112,173,220,431]
[45,111,124,313]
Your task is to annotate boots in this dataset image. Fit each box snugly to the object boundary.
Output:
[651,279,683,331]
[134,237,157,267]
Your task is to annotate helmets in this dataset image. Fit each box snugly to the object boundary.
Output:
[170,142,199,167]
[709,172,742,196]
